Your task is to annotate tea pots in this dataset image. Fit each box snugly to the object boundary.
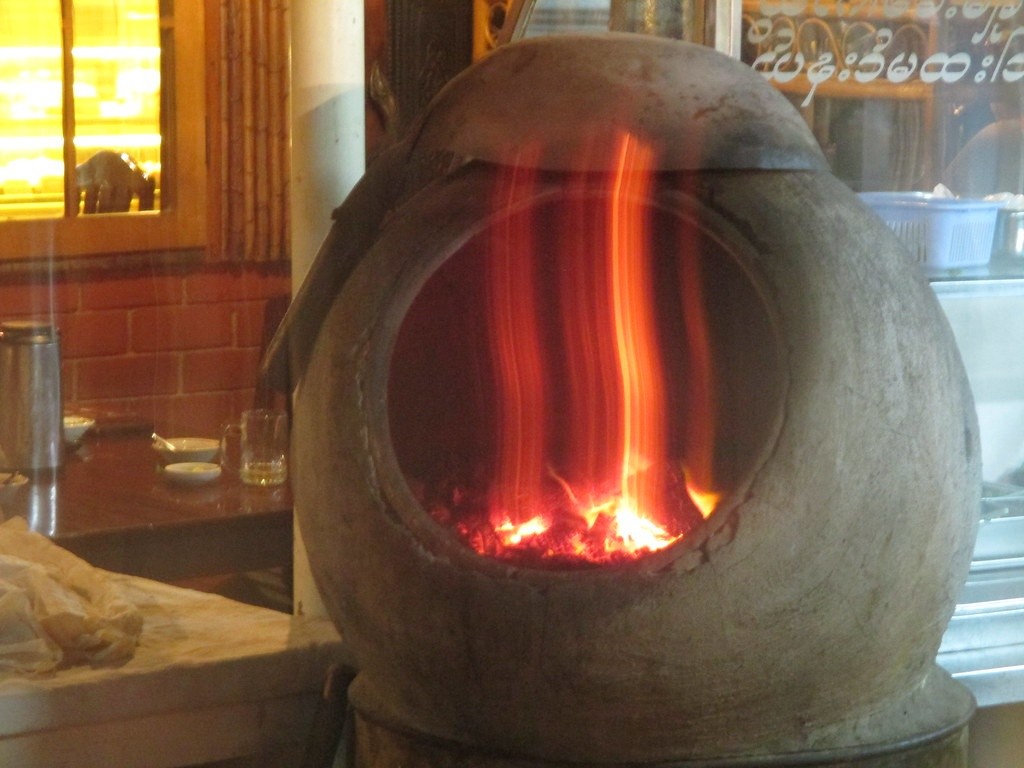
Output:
[0,318,63,470]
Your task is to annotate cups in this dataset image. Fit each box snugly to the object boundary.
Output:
[218,409,290,485]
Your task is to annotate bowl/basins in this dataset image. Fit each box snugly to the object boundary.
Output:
[63,415,96,443]
[164,461,222,485]
[0,473,28,505]
[152,436,220,462]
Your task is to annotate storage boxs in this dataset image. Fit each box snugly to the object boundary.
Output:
[860,187,1003,271]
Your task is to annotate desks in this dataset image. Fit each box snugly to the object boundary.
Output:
[0,409,294,583]
